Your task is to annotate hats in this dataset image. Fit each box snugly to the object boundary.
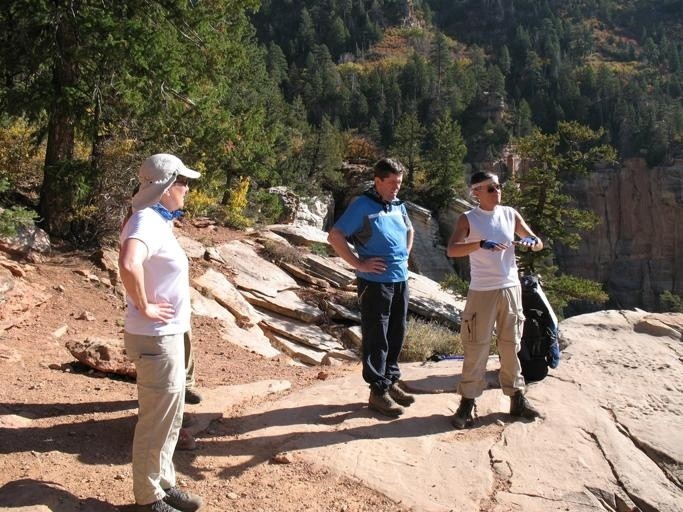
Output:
[132,152,201,210]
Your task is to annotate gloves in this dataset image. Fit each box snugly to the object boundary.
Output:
[479,238,504,250]
[519,236,538,247]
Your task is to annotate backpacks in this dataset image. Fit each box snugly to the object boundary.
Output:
[516,274,560,385]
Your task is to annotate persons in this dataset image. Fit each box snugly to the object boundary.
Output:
[120,182,201,452]
[447,170,543,428]
[119,153,206,512]
[327,155,416,416]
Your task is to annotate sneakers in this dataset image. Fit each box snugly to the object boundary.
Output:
[451,395,474,429]
[185,385,202,404]
[509,391,546,421]
[388,381,415,405]
[133,501,182,512]
[368,388,405,417]
[182,412,196,428]
[163,486,203,512]
[176,429,197,450]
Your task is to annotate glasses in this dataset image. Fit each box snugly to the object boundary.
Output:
[174,180,188,187]
[478,184,502,193]
